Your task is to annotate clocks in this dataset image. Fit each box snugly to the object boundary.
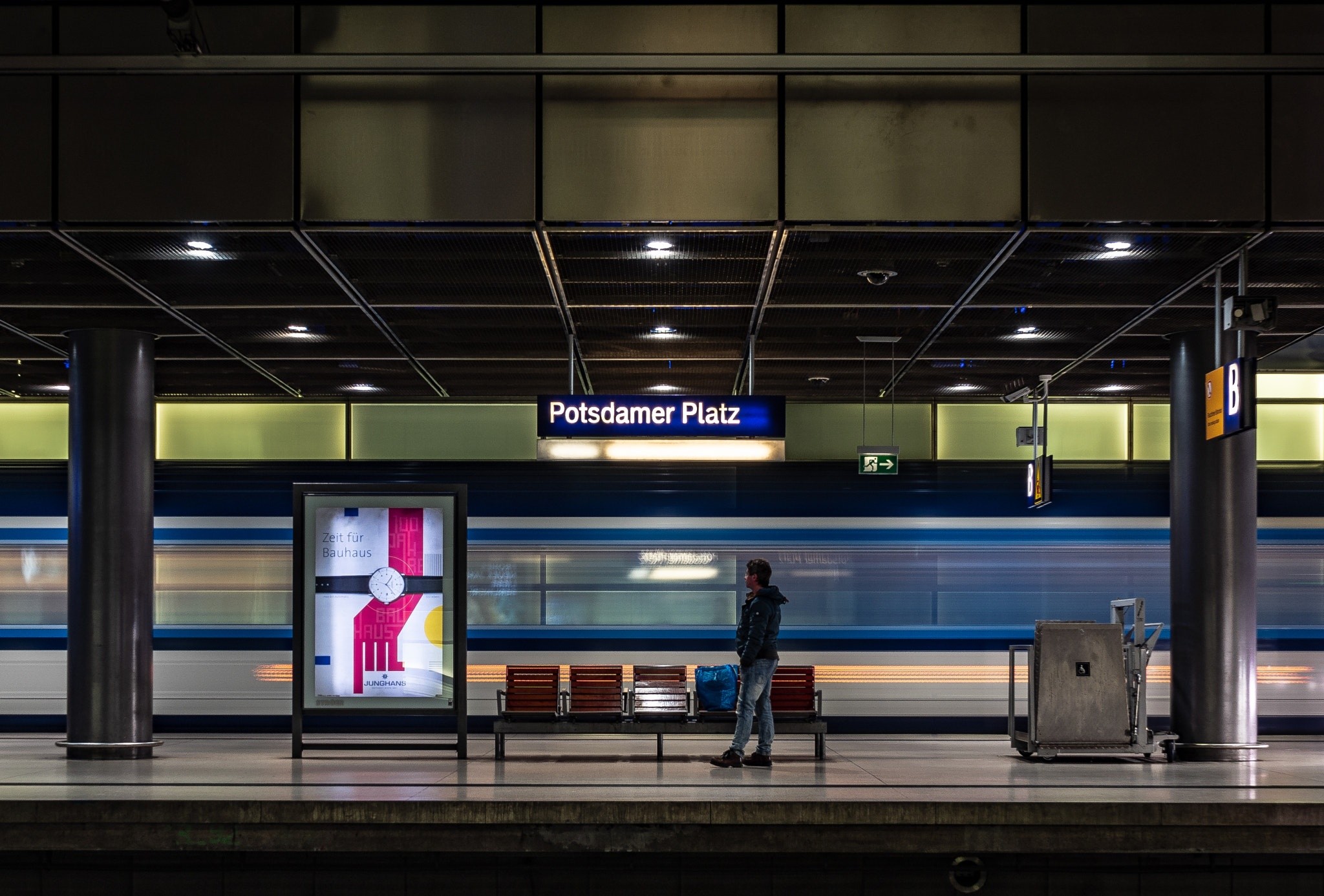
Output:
[369,569,407,605]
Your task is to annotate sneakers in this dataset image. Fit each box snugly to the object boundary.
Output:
[742,752,774,767]
[708,748,745,768]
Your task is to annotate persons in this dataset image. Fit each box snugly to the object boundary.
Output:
[709,559,789,768]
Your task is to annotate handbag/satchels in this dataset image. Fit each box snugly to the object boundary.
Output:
[695,663,739,712]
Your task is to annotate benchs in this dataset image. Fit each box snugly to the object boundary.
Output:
[494,665,829,766]
